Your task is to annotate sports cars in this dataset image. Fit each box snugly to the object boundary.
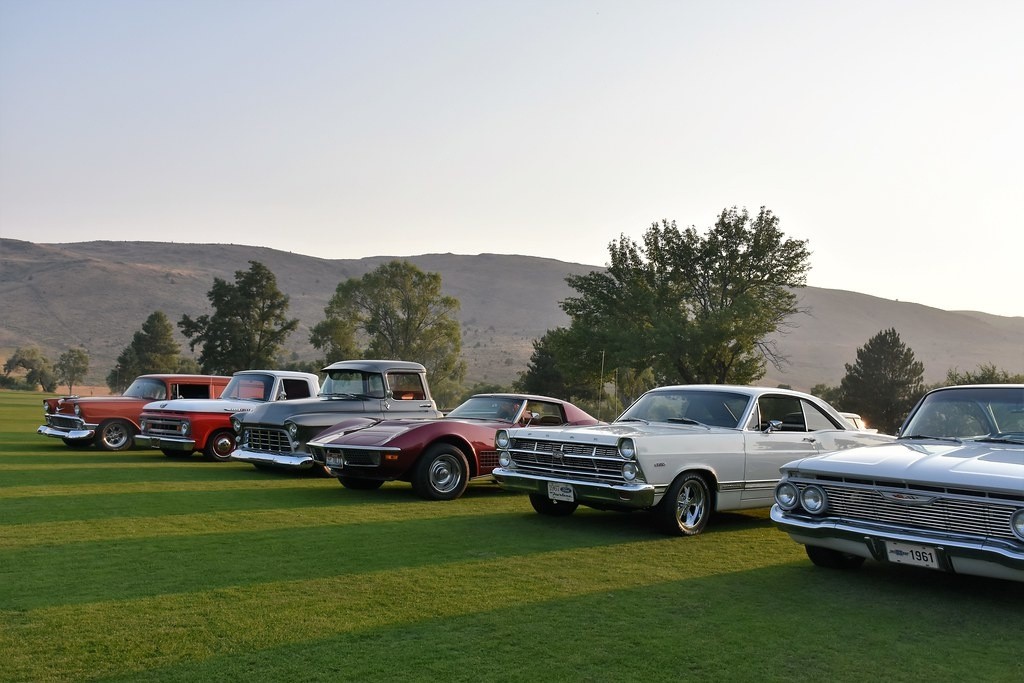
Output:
[304,392,610,501]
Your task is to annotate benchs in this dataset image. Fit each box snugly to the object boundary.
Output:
[368,390,423,400]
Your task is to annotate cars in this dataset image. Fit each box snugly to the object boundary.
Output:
[37,372,265,453]
[133,369,321,463]
[781,410,865,431]
[491,383,897,537]
[227,359,445,479]
[770,383,1024,587]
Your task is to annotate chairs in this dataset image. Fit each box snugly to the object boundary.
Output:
[539,416,561,426]
[781,411,805,431]
